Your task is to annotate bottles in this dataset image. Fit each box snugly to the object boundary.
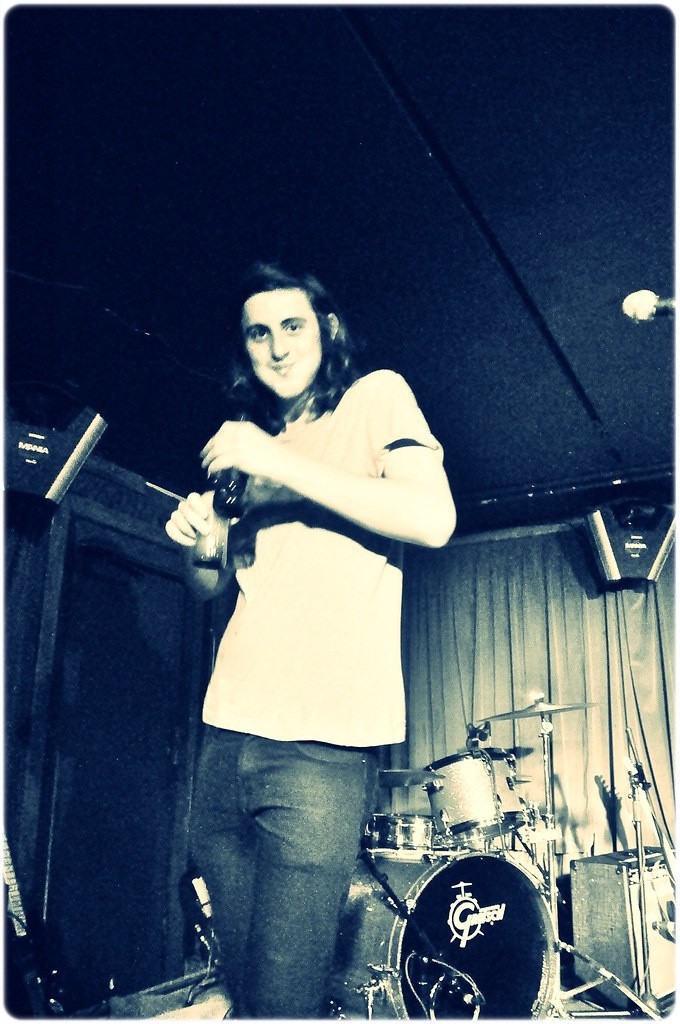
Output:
[212,409,253,518]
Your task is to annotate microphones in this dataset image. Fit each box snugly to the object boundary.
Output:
[462,994,486,1005]
[651,921,676,931]
[479,720,490,741]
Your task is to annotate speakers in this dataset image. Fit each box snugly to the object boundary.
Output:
[571,846,678,1011]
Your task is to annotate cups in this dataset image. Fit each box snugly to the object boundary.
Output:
[192,489,231,570]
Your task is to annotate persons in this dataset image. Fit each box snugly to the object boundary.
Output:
[163,252,457,1021]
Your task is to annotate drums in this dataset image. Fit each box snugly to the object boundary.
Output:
[385,852,555,1021]
[365,812,436,864]
[423,747,527,850]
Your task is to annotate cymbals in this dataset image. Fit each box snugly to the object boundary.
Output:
[436,820,527,848]
[378,767,447,789]
[475,700,601,724]
